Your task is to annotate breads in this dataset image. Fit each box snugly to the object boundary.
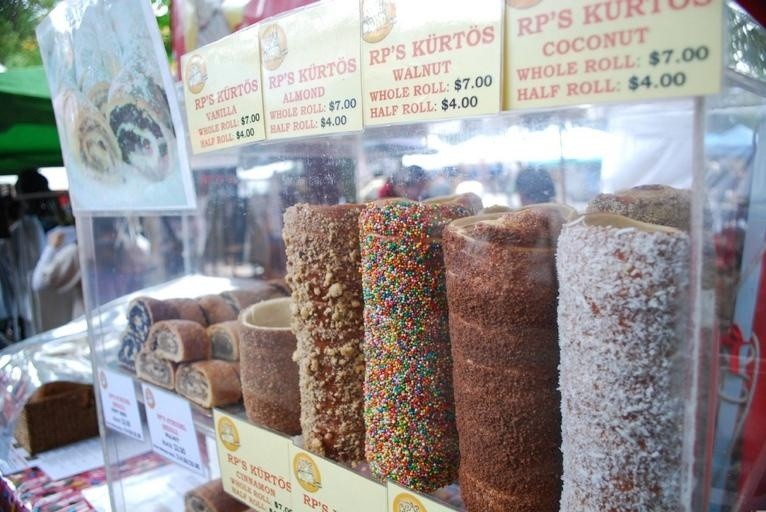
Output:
[197,295,237,323]
[271,279,292,295]
[425,192,481,213]
[224,288,290,316]
[238,298,300,435]
[556,213,689,512]
[175,358,240,409]
[136,349,175,390]
[118,332,143,370]
[183,478,250,512]
[443,206,566,512]
[54,70,176,183]
[282,203,365,464]
[481,206,512,214]
[166,298,206,325]
[359,199,471,493]
[584,185,715,512]
[126,297,179,340]
[527,203,578,220]
[27,383,73,401]
[206,320,239,360]
[231,361,240,377]
[146,319,209,362]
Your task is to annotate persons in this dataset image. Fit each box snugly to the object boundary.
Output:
[32,220,117,319]
[376,165,430,203]
[515,167,557,206]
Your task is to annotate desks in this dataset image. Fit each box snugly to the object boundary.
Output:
[0,414,161,512]
